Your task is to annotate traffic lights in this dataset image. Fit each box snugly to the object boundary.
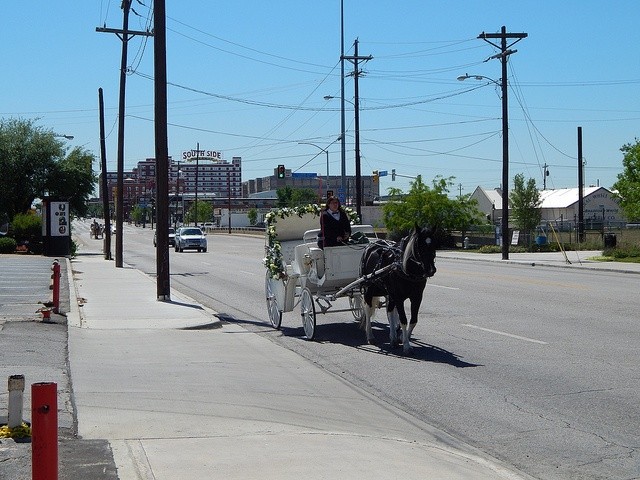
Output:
[278,165,285,178]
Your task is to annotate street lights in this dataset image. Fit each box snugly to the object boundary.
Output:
[458,72,508,259]
[323,96,362,225]
[41,134,74,201]
[297,141,330,192]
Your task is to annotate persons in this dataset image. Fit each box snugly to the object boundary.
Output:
[317,195,351,251]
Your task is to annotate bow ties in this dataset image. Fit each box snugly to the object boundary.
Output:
[332,211,338,215]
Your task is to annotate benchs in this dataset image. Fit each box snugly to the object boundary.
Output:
[303,225,378,248]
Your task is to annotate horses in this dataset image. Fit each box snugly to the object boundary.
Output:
[358,219,439,357]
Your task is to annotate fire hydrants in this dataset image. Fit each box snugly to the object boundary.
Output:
[464,237,469,250]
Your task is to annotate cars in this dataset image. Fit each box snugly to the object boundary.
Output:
[154,228,175,247]
[112,226,116,235]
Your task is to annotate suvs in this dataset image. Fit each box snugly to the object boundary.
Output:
[175,227,207,253]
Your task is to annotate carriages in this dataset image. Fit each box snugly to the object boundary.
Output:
[263,204,438,358]
[90,223,103,240]
